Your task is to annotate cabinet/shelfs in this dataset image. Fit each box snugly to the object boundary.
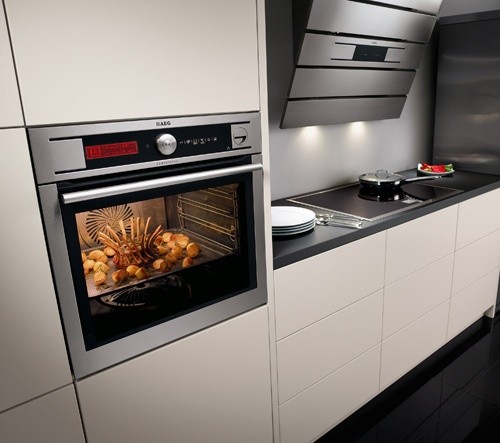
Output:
[72,303,276,443]
[1,0,274,127]
[0,381,87,442]
[1,125,76,424]
[381,198,461,394]
[446,186,500,346]
[272,225,386,442]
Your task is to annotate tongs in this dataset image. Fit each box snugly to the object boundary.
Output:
[314,211,364,230]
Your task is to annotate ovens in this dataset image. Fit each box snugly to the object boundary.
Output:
[24,108,268,382]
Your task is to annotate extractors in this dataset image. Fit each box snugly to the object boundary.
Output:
[280,1,443,130]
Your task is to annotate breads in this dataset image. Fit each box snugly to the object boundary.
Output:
[81,217,199,285]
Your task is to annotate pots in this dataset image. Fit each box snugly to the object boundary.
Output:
[358,168,442,191]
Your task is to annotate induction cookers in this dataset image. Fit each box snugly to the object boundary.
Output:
[286,175,465,221]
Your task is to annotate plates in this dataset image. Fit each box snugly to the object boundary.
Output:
[270,204,317,237]
[417,164,456,176]
